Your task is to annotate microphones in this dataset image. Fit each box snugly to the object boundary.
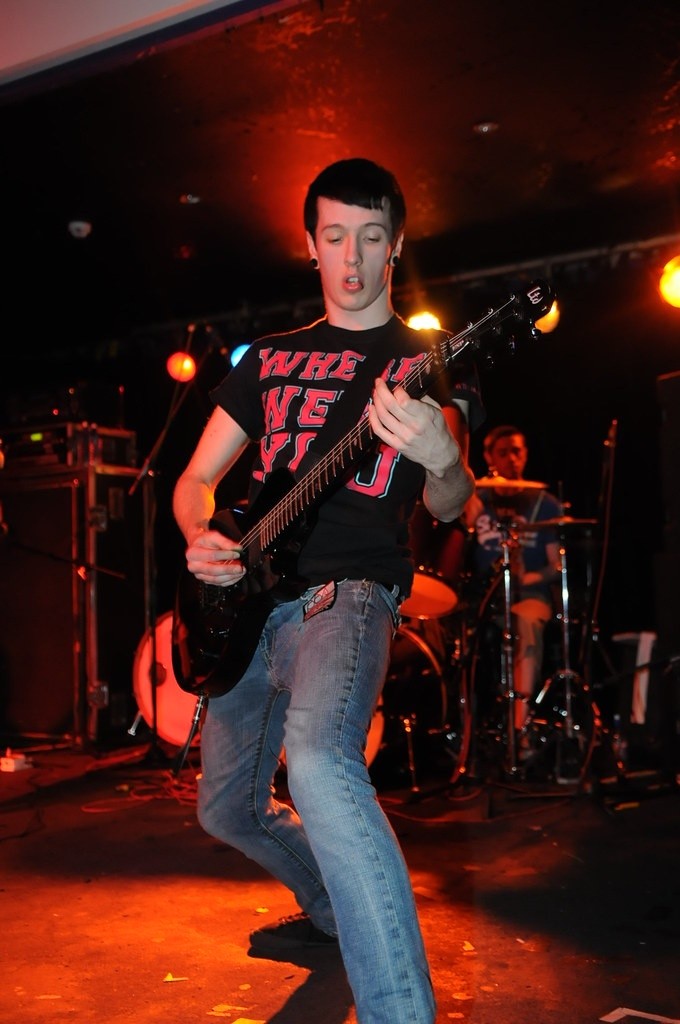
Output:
[203,325,232,364]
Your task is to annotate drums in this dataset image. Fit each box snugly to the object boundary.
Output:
[395,493,484,623]
[360,624,452,792]
[129,611,208,752]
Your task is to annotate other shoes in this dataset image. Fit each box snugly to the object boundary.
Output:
[248,907,338,946]
[516,730,537,760]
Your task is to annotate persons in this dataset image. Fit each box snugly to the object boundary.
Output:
[167,158,487,1024]
[433,425,571,766]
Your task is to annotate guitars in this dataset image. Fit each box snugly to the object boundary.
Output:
[170,271,557,701]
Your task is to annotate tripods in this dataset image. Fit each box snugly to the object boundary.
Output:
[404,490,670,826]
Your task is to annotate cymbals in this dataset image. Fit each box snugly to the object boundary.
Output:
[513,514,605,535]
[470,474,549,494]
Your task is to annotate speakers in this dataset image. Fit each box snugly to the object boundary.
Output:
[0,479,85,738]
